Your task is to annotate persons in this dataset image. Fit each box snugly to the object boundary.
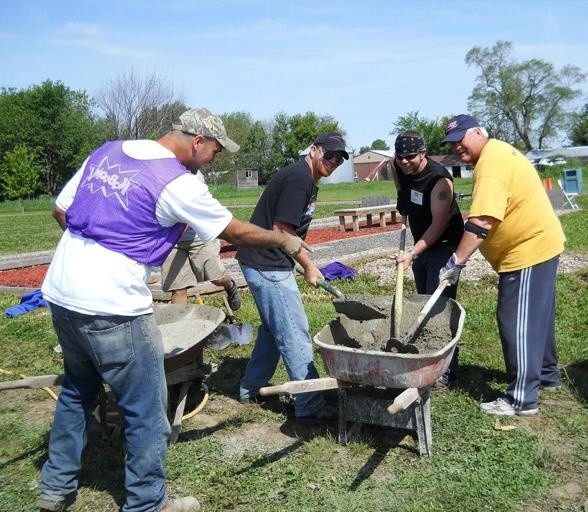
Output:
[34,106,315,512]
[437,113,565,418]
[391,129,464,389]
[162,168,241,309]
[235,132,349,422]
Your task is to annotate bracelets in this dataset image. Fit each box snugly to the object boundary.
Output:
[408,247,418,267]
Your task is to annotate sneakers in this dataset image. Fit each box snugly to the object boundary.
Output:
[295,401,339,422]
[480,397,538,416]
[240,383,290,404]
[160,496,200,512]
[38,490,81,512]
[540,385,562,392]
[224,279,241,311]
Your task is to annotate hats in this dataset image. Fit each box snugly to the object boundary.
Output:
[440,114,480,147]
[172,107,241,153]
[314,132,349,160]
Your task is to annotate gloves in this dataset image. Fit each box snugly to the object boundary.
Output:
[438,252,466,287]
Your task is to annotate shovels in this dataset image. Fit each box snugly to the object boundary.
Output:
[221,294,253,345]
[290,255,387,321]
[189,287,232,351]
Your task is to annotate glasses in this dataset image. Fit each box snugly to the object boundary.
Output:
[395,153,417,160]
[324,151,344,166]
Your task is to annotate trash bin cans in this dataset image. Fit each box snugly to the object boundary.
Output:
[562,166,583,194]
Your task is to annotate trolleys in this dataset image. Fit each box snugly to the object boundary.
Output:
[11,298,229,446]
[314,282,469,455]
